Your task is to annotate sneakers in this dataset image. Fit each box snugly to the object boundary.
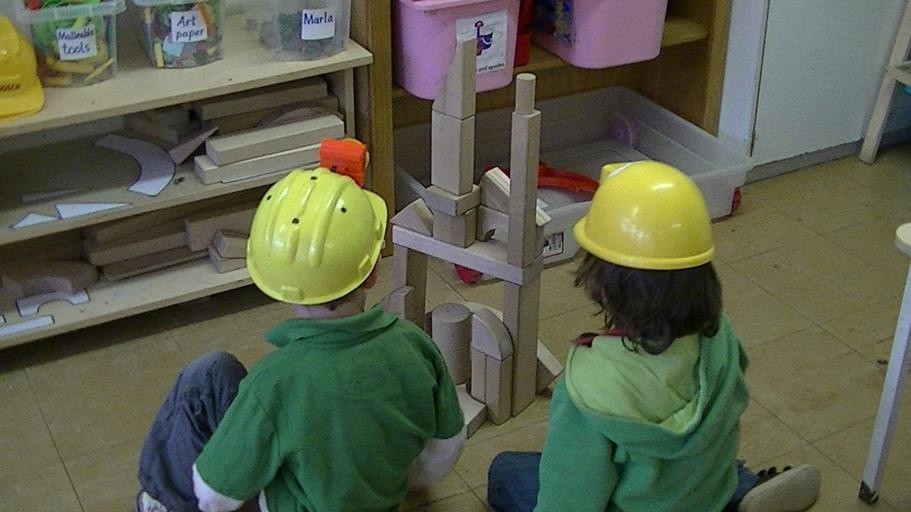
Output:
[137,490,168,512]
[739,464,820,511]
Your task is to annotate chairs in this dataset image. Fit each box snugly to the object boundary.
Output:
[858,224,907,504]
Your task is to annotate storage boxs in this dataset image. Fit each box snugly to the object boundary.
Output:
[13,1,128,88]
[393,0,520,101]
[529,0,668,69]
[133,1,223,69]
[391,85,754,289]
[239,0,353,61]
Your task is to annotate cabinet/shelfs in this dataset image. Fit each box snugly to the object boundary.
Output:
[350,0,732,257]
[717,1,911,186]
[0,10,373,355]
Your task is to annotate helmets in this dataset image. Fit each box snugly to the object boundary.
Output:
[573,160,713,271]
[247,167,388,305]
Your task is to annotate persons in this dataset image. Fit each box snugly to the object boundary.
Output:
[487,159,822,512]
[137,134,466,512]
